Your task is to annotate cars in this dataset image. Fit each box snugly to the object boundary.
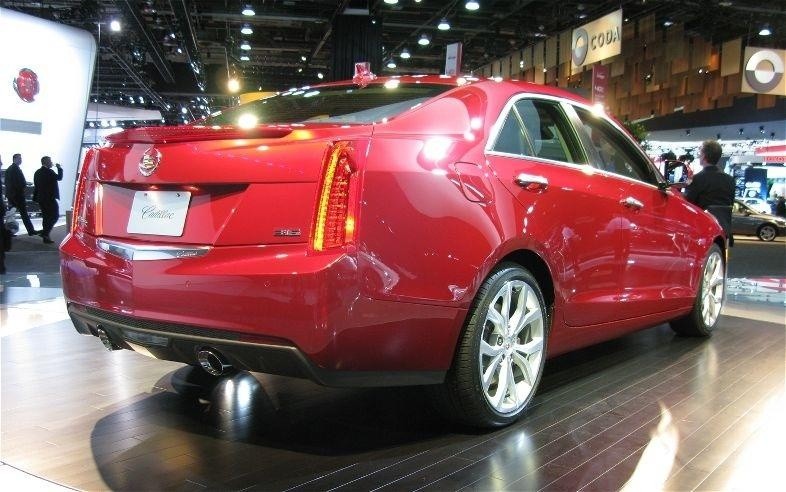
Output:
[728,199,785,244]
[57,59,731,432]
[0,168,42,218]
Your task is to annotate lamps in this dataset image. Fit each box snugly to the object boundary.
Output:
[717,134,722,143]
[757,18,772,37]
[382,0,480,69]
[238,1,256,61]
[686,130,690,137]
[738,128,744,136]
[759,126,765,134]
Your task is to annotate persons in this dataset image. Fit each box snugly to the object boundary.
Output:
[33,157,63,243]
[684,138,734,229]
[5,155,43,236]
